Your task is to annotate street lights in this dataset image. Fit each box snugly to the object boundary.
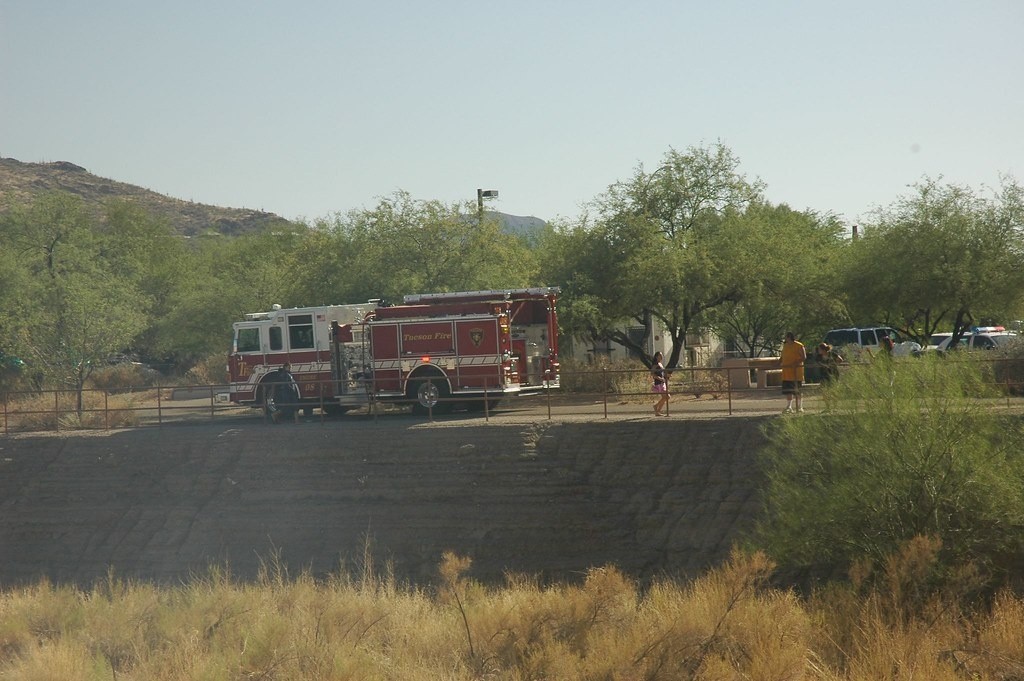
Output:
[477,188,499,229]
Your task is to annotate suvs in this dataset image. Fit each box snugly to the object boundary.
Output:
[822,326,921,360]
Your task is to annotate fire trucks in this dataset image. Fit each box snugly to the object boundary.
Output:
[224,289,561,416]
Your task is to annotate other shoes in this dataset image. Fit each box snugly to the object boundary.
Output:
[782,407,794,414]
[655,412,663,416]
[653,405,659,413]
[798,407,804,412]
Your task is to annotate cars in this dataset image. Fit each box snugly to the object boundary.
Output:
[936,327,1021,353]
[922,333,955,352]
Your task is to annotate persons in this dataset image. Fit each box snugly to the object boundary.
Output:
[867,336,896,386]
[778,332,807,415]
[815,343,843,415]
[650,351,671,416]
[270,361,301,424]
